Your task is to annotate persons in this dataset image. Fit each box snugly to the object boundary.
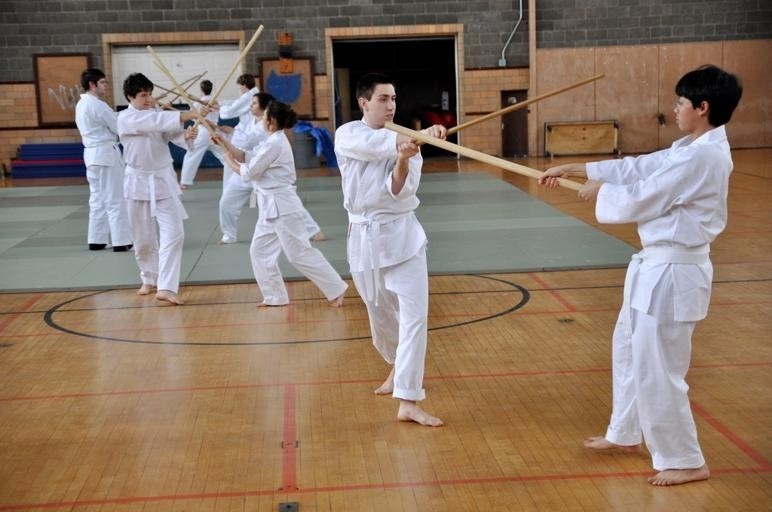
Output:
[210,74,260,192]
[75,67,132,253]
[175,79,228,190]
[211,98,349,308]
[335,75,446,427]
[116,73,213,305]
[207,93,327,244]
[538,66,744,487]
